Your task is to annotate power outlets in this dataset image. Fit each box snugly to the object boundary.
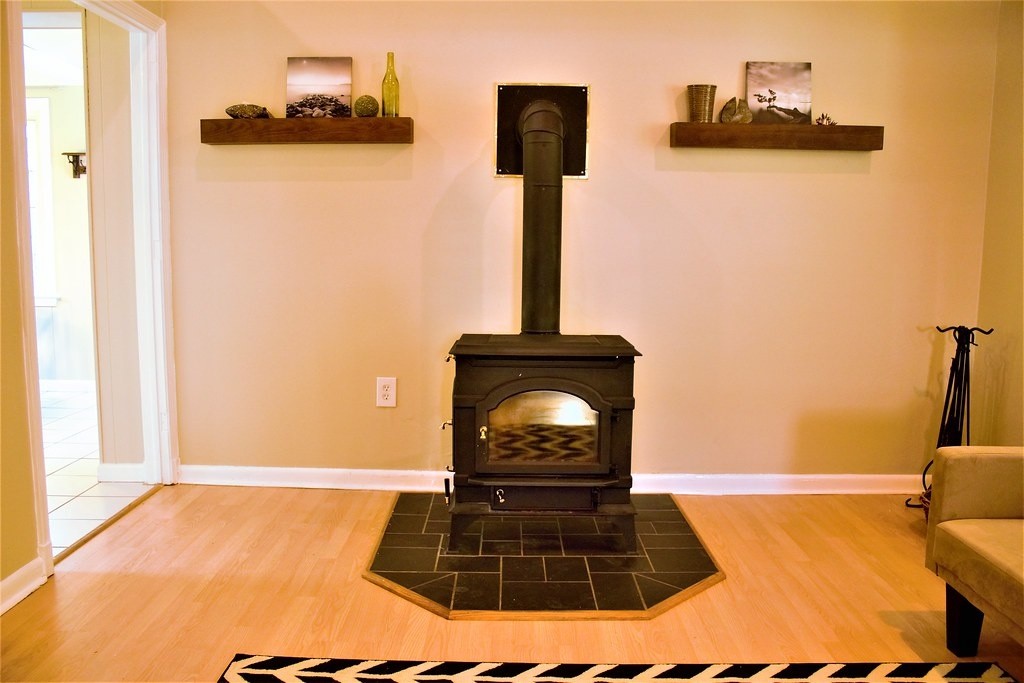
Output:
[376,377,396,407]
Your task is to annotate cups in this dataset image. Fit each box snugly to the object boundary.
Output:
[687,85,717,123]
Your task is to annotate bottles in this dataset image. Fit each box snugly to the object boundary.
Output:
[382,52,399,118]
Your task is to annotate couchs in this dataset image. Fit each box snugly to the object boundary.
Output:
[925,445,1024,658]
